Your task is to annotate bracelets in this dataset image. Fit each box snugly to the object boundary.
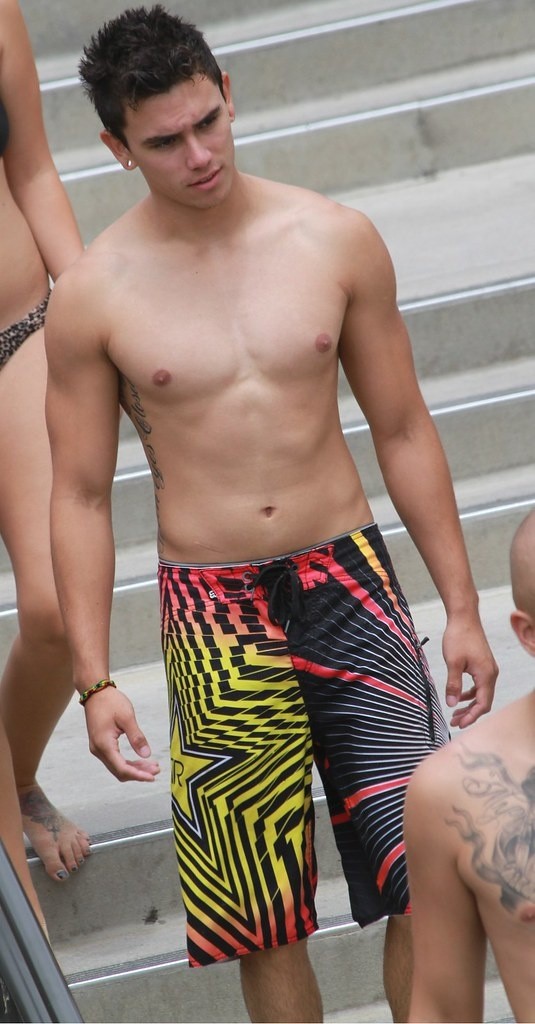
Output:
[79,679,115,706]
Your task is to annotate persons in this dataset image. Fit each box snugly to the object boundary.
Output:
[0,0,93,881]
[403,506,535,1024]
[43,6,500,1023]
